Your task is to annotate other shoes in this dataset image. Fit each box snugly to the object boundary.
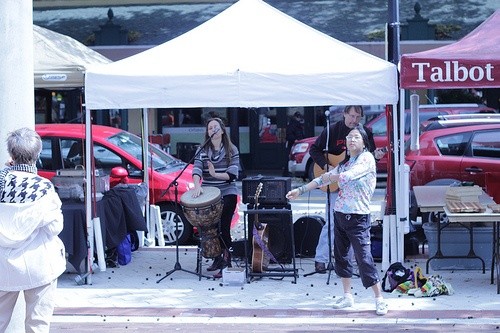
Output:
[207,258,222,271]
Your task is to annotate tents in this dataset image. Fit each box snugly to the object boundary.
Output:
[400,7,500,266]
[33,24,147,285]
[85,0,400,283]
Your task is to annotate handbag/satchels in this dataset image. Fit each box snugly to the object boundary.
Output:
[117,234,131,265]
[382,262,410,292]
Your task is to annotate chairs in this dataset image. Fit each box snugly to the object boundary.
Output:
[64,142,81,169]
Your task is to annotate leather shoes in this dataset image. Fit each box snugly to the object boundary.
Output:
[315,261,325,273]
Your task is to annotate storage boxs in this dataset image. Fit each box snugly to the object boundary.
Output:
[422,223,497,270]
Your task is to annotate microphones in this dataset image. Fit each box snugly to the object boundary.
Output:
[325,110,330,120]
[214,127,221,134]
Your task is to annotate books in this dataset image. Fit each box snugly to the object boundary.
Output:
[446,187,500,213]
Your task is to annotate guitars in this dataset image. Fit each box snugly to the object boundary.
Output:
[313,145,388,193]
[252,183,268,273]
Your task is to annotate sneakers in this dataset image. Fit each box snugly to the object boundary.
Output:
[332,295,354,309]
[376,300,388,315]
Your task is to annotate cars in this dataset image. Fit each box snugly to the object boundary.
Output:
[35,124,242,245]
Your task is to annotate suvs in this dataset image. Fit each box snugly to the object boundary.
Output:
[382,113,500,205]
[288,103,500,189]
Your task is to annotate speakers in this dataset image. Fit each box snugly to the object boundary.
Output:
[247,202,293,263]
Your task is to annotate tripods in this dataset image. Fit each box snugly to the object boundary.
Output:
[156,134,215,284]
[303,118,360,285]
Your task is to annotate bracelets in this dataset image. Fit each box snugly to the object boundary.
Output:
[298,185,306,195]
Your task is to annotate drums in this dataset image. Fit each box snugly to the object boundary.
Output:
[180,187,224,259]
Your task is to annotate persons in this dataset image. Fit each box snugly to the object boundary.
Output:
[286,112,305,149]
[309,105,375,274]
[192,117,239,272]
[286,126,387,315]
[0,128,67,333]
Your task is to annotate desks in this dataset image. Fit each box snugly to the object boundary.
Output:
[413,185,500,294]
[58,184,146,285]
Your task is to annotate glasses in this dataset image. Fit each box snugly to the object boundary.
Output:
[346,136,362,141]
